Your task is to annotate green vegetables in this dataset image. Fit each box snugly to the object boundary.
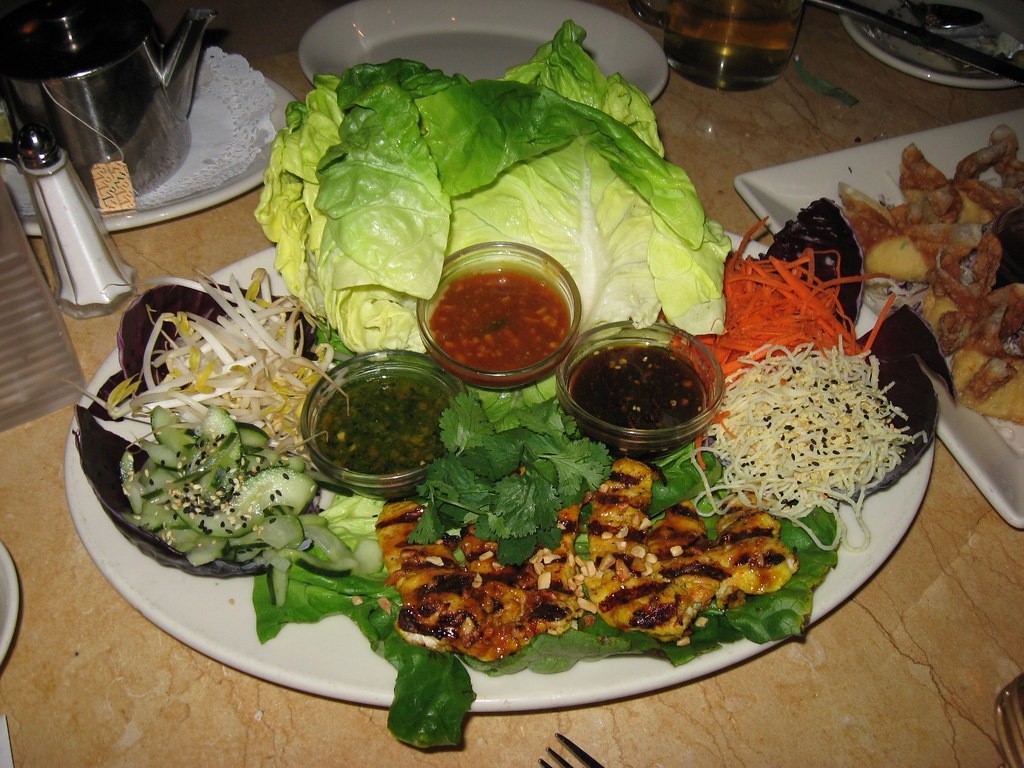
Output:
[256,16,730,552]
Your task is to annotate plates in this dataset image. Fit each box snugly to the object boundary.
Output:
[66,246,936,711]
[0,540,19,668]
[27,77,307,236]
[733,109,1024,532]
[839,0,1024,89]
[300,0,668,123]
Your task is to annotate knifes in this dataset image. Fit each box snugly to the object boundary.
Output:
[808,0,1024,83]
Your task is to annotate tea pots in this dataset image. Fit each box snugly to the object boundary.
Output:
[0,0,216,212]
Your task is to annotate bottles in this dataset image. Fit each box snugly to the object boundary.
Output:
[18,125,137,319]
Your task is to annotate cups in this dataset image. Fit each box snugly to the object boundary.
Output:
[995,674,1024,768]
[628,0,804,90]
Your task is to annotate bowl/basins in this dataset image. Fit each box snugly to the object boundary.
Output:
[555,320,728,462]
[301,350,467,499]
[417,241,581,389]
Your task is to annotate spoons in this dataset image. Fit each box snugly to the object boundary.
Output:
[898,0,984,29]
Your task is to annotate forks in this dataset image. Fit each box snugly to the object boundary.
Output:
[538,734,603,768]
